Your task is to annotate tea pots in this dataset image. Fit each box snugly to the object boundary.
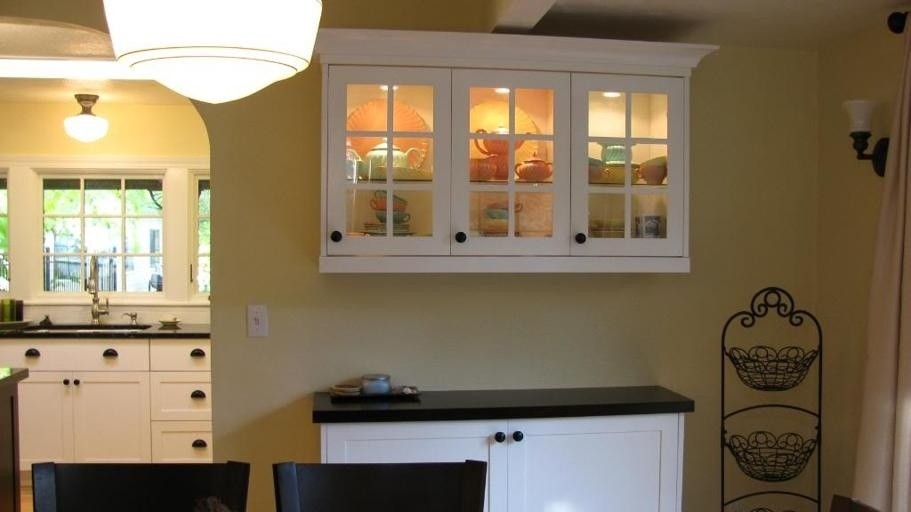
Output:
[473,124,554,182]
[347,137,422,180]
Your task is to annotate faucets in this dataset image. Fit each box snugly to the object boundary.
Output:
[88,255,111,327]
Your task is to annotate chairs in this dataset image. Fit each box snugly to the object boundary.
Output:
[272,464,485,511]
[31,464,255,511]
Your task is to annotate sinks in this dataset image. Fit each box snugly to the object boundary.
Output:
[23,325,153,333]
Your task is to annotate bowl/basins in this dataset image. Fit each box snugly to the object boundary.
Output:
[589,216,625,229]
[588,158,602,182]
[640,156,667,185]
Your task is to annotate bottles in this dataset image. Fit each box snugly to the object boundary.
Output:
[361,374,391,395]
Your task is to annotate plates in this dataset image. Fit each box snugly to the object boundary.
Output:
[360,223,416,235]
[157,319,181,326]
[588,227,625,238]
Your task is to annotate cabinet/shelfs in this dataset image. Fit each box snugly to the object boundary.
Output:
[320,30,450,273]
[570,37,692,273]
[320,415,682,511]
[446,30,571,272]
[2,339,212,485]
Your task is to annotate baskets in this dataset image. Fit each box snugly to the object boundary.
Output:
[729,430,817,484]
[725,343,817,392]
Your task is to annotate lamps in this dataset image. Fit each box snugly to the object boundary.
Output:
[839,98,890,179]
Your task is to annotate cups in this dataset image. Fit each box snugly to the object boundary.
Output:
[480,201,523,238]
[634,213,666,237]
[370,189,411,223]
